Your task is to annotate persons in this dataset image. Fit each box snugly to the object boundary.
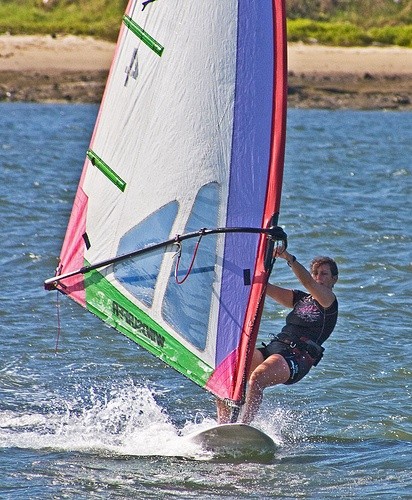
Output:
[217,246,340,424]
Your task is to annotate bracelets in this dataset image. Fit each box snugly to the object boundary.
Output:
[286,255,296,267]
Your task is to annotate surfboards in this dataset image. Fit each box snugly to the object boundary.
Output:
[185,423,278,451]
